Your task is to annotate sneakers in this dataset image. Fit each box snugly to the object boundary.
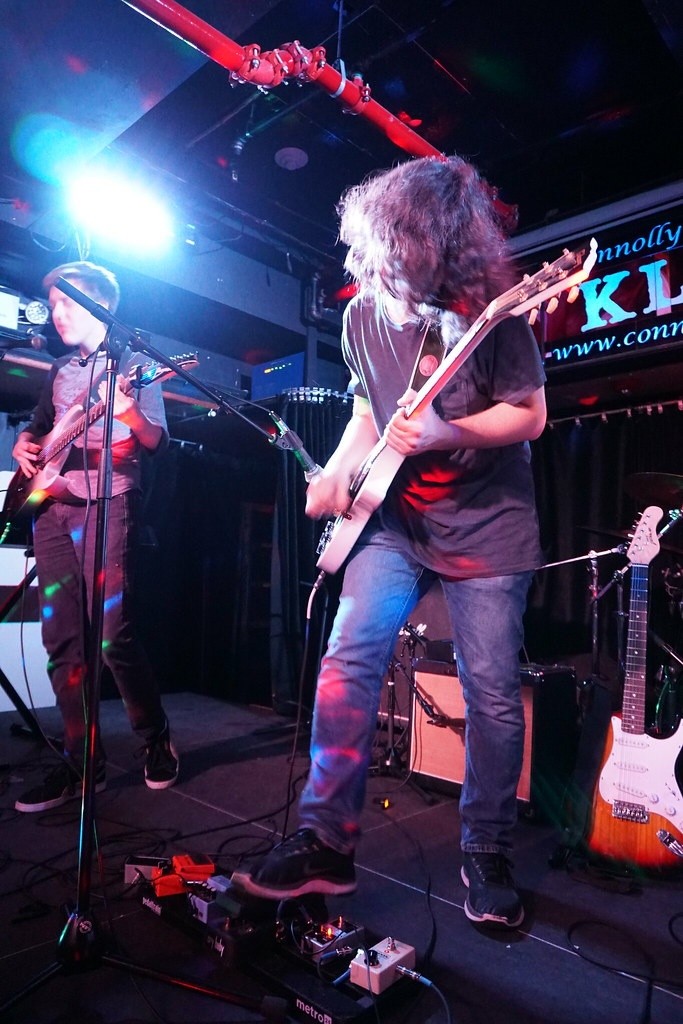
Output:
[231,827,359,899]
[143,725,180,788]
[461,850,523,927]
[16,758,106,813]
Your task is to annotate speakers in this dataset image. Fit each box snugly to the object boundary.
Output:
[406,658,580,807]
[377,580,459,729]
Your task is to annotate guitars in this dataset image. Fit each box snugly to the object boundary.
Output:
[1,349,202,532]
[312,233,602,577]
[581,503,683,878]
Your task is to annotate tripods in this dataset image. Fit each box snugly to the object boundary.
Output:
[0,277,291,1024]
[370,651,445,807]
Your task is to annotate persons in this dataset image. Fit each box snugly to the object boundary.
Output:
[12,261,179,812]
[231,158,547,926]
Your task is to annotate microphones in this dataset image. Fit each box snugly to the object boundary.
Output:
[269,411,324,482]
[432,718,467,729]
[0,335,48,351]
